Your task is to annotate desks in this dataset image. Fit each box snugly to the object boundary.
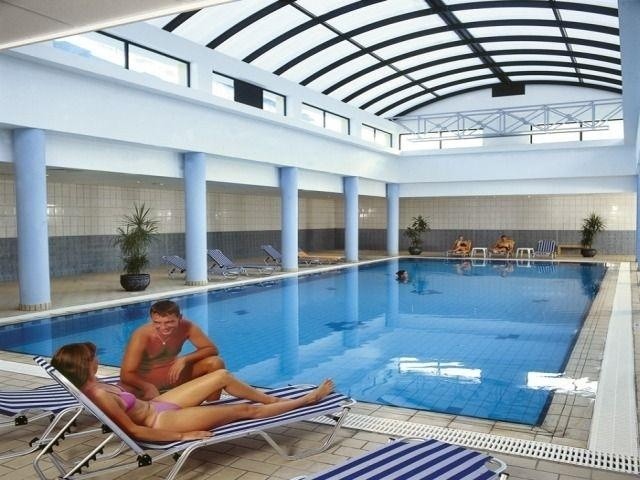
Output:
[471,260,487,267]
[516,260,534,268]
[516,248,534,259]
[471,247,488,258]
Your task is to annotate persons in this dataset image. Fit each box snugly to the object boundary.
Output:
[496,261,513,278]
[453,234,470,254]
[50,341,336,444]
[455,261,469,275]
[117,300,224,404]
[493,236,513,254]
[396,269,409,281]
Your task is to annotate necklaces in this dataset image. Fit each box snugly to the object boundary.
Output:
[155,332,173,346]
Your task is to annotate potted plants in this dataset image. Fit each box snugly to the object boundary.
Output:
[578,210,608,256]
[112,201,159,290]
[402,214,432,255]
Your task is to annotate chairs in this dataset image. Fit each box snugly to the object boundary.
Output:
[161,255,239,280]
[287,435,509,480]
[447,236,471,258]
[33,355,358,480]
[0,375,121,459]
[488,235,515,258]
[262,243,345,268]
[534,239,557,259]
[208,249,280,277]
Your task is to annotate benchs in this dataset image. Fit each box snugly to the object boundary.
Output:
[558,244,589,255]
